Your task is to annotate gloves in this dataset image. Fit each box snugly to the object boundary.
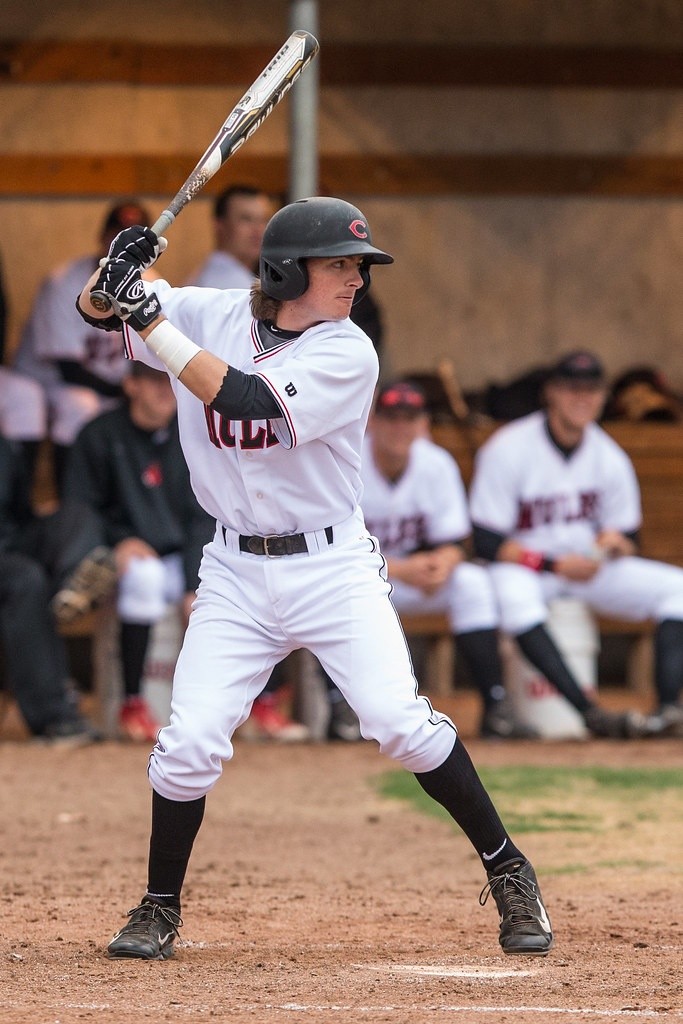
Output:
[108,225,169,273]
[91,258,162,331]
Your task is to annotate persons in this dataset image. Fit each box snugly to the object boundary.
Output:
[336,285,683,426]
[469,350,683,741]
[322,382,540,741]
[179,181,282,294]
[59,361,312,744]
[75,197,553,961]
[17,199,168,509]
[0,258,100,747]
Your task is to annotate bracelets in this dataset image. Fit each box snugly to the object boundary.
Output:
[144,320,204,380]
[519,550,543,571]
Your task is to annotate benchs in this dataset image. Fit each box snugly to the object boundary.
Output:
[0,423,682,743]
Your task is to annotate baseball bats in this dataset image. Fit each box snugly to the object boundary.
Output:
[89,28,321,313]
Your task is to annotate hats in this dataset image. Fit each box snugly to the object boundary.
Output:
[377,379,430,420]
[552,348,609,396]
[97,202,152,245]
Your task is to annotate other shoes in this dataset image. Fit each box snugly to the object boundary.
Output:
[111,700,156,744]
[41,715,102,745]
[239,692,310,744]
[588,705,645,741]
[489,710,541,744]
[656,702,683,730]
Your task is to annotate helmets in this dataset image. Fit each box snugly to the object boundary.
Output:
[260,196,394,307]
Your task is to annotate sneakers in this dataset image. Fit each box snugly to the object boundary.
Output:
[108,894,181,961]
[481,859,553,951]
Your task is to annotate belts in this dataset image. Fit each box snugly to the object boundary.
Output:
[223,527,333,555]
